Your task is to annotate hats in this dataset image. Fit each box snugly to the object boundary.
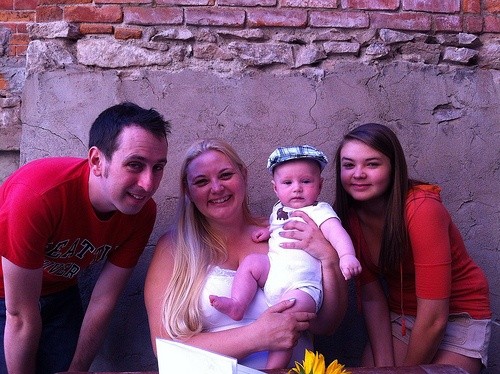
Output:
[267,145,328,177]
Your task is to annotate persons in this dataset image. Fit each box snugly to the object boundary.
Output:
[144,138,348,374]
[331,122,491,374]
[0,102,172,374]
[209,144,362,369]
